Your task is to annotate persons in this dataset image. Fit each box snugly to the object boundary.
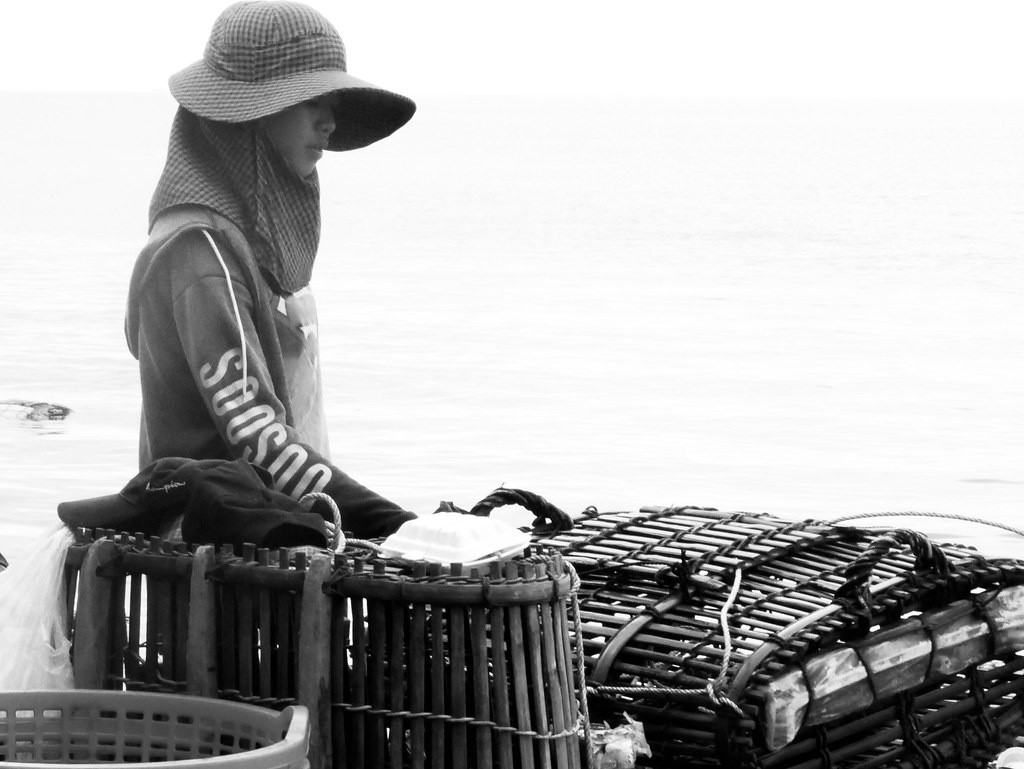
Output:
[124,0,419,660]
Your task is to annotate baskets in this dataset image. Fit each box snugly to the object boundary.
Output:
[0,689,310,769]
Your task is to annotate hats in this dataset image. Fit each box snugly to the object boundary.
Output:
[168,2,416,152]
[57,457,198,540]
[180,461,328,552]
